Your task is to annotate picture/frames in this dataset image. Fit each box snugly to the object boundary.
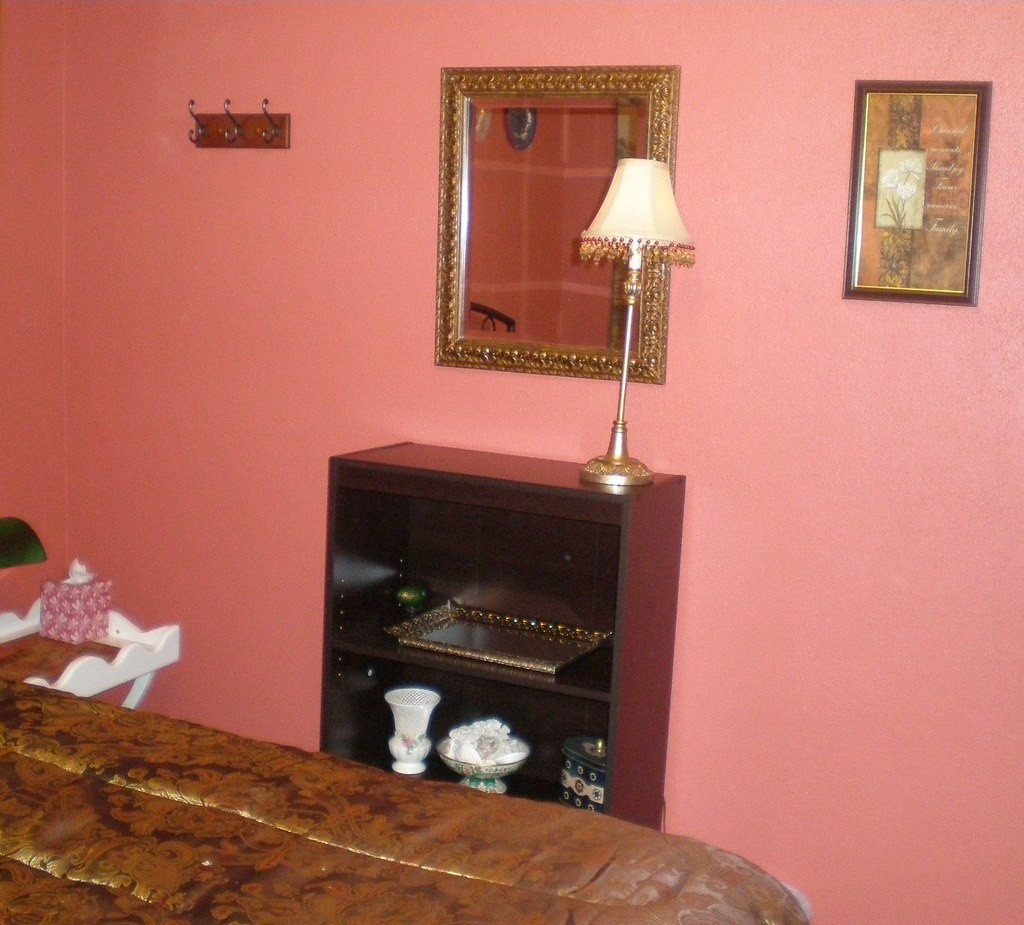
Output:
[844,79,994,306]
[383,601,613,674]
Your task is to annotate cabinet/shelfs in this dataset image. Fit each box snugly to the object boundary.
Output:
[319,441,686,829]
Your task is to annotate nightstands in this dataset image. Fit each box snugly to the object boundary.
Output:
[0,598,179,709]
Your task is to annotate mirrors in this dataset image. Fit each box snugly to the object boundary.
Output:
[436,64,679,385]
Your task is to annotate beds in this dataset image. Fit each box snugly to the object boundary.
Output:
[0,671,812,925]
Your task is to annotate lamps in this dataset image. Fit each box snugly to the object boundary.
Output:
[579,159,695,487]
